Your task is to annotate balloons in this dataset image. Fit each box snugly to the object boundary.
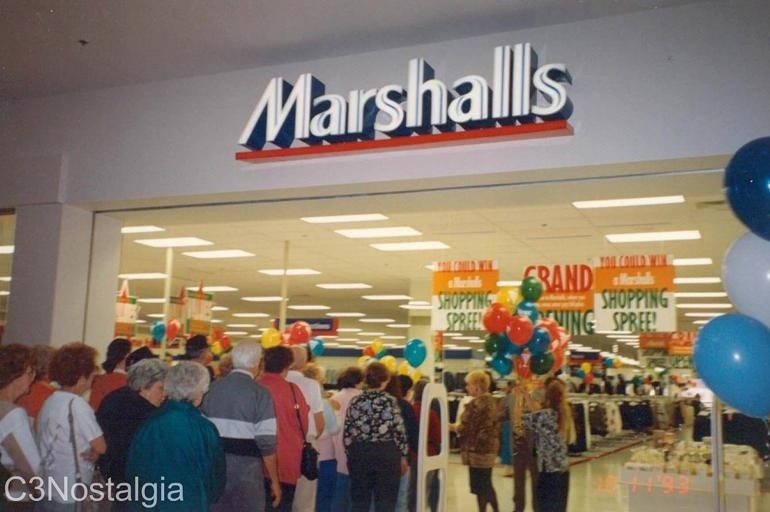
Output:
[262,327,284,349]
[479,276,566,381]
[581,361,592,372]
[165,320,182,338]
[209,321,226,336]
[613,357,622,369]
[691,312,770,422]
[151,321,165,342]
[575,367,582,376]
[287,321,310,344]
[722,136,770,243]
[605,358,613,367]
[721,232,770,332]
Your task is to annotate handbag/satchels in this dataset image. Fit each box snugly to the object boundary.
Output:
[301,442,320,482]
[363,439,401,476]
[72,469,116,512]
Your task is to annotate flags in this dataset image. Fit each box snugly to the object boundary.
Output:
[638,332,671,359]
[432,260,500,334]
[668,330,696,356]
[593,263,677,335]
[521,266,595,335]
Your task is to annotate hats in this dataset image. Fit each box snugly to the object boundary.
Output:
[185,334,215,351]
[125,345,161,366]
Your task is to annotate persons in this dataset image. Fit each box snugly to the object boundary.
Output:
[494,378,545,512]
[0,335,443,511]
[568,373,703,417]
[448,371,501,511]
[512,379,572,511]
